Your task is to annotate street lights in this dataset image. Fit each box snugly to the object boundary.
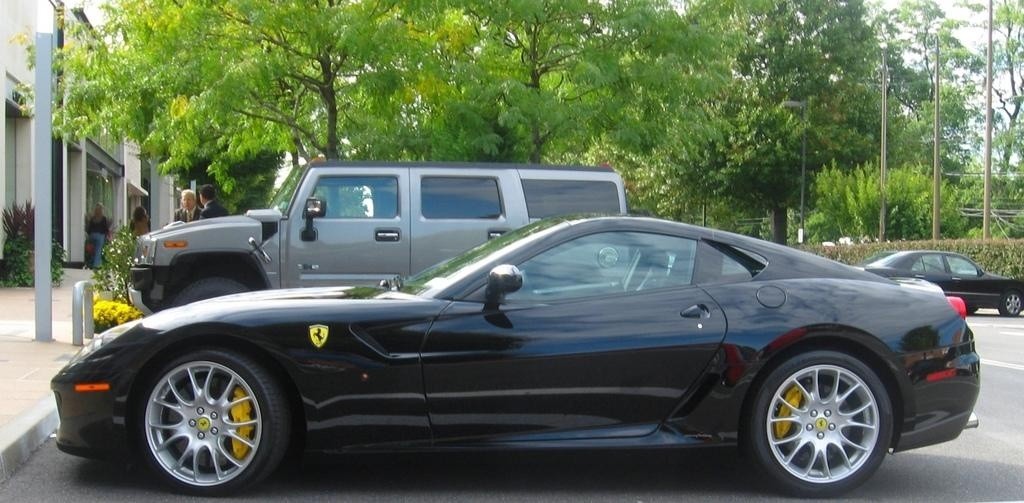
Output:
[931,23,942,239]
[878,42,894,241]
[783,99,808,242]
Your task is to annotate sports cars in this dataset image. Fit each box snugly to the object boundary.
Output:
[47,214,980,498]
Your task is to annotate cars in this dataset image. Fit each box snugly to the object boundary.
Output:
[289,345,388,383]
[858,249,1024,317]
[906,349,978,383]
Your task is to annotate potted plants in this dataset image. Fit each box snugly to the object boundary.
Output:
[2,236,68,292]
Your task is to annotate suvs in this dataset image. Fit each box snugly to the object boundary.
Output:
[126,164,627,319]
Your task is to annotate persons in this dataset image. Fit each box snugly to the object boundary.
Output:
[130,206,150,241]
[174,189,202,222]
[88,202,111,270]
[198,184,229,219]
